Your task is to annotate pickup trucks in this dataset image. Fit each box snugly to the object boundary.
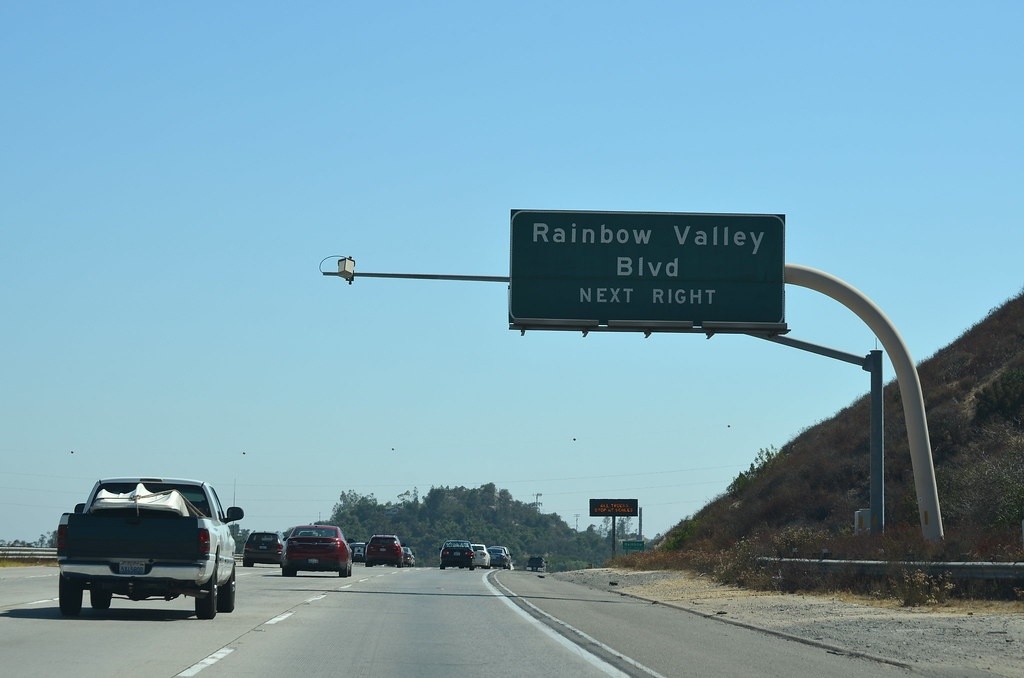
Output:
[57,476,245,620]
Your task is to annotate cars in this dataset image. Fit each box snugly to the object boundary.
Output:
[439,540,477,570]
[487,548,509,569]
[470,543,492,569]
[349,541,368,564]
[401,546,415,568]
[282,524,355,578]
[526,557,548,574]
[365,534,404,569]
[490,545,513,569]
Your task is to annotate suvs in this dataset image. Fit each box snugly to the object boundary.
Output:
[242,530,284,568]
